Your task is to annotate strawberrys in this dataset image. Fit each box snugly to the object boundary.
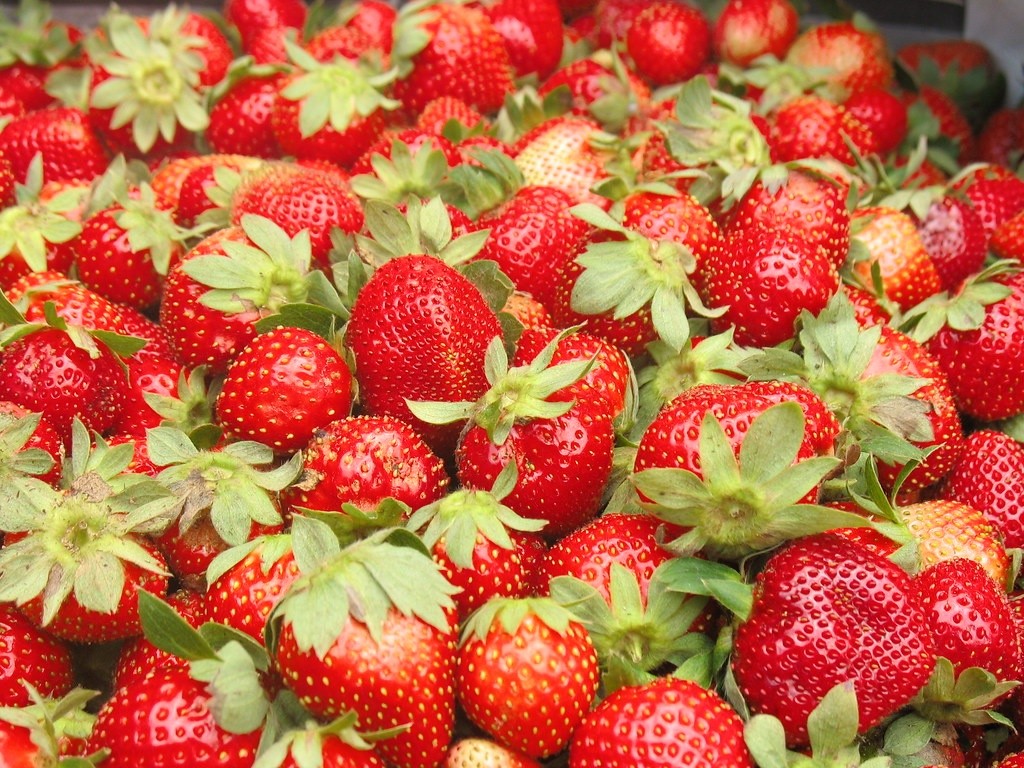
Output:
[0,0,1024,768]
